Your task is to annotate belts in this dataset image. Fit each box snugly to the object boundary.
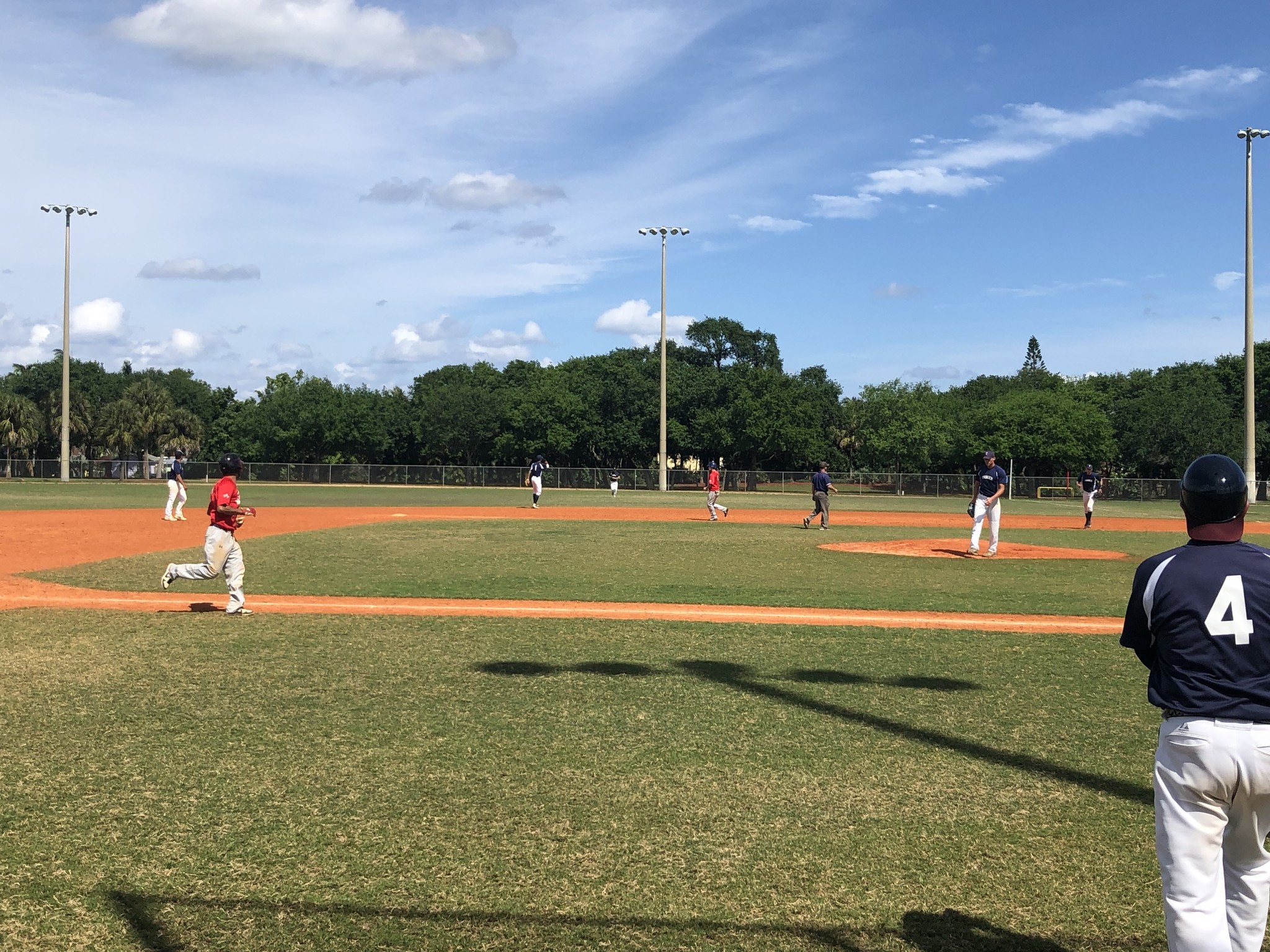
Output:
[1164,708,1270,725]
[979,492,991,497]
[1084,490,1094,492]
[214,524,234,532]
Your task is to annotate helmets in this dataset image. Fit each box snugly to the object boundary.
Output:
[1180,454,1248,523]
[220,453,241,475]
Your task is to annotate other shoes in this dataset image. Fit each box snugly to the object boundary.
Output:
[983,551,997,557]
[165,514,187,521]
[709,516,718,520]
[536,504,539,506]
[533,503,539,508]
[1084,521,1091,528]
[819,526,831,530]
[968,547,978,555]
[724,509,728,517]
[804,518,810,529]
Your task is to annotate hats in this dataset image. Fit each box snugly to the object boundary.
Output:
[175,450,185,456]
[613,467,616,470]
[820,462,829,467]
[707,462,716,469]
[1086,464,1092,469]
[983,451,995,459]
[1187,513,1244,542]
[537,455,543,460]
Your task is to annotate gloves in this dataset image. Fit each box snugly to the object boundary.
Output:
[236,508,256,527]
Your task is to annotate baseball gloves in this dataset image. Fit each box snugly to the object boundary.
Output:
[967,502,975,518]
[524,479,530,486]
[235,517,245,528]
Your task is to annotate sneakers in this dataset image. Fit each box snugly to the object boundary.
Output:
[227,607,253,615]
[161,563,173,590]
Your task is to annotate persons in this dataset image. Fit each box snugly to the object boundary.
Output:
[525,454,550,509]
[608,467,621,498]
[803,462,838,531]
[160,453,255,615]
[703,461,729,521]
[1076,463,1103,529]
[1119,453,1270,952]
[966,450,1006,558]
[163,450,189,522]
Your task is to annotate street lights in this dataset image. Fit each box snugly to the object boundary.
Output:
[639,227,690,491]
[40,204,97,482]
[1236,125,1270,504]
